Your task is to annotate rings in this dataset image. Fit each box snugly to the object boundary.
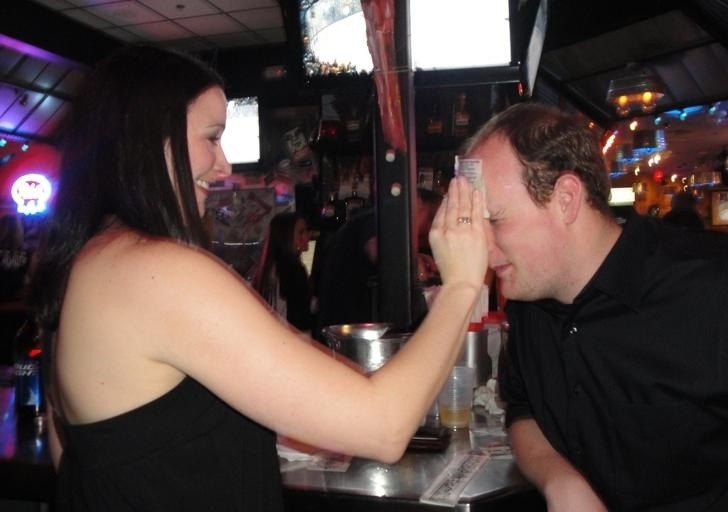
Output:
[457,217,470,224]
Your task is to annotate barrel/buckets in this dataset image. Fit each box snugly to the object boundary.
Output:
[321,323,416,431]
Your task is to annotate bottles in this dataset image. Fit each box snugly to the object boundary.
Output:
[315,89,374,227]
[417,90,474,202]
[11,294,51,416]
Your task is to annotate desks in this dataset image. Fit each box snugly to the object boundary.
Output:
[276,411,591,511]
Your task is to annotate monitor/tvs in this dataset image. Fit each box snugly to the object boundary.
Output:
[225,94,274,172]
[409,2,523,88]
[287,2,377,90]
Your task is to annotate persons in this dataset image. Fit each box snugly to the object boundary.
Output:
[258,209,313,333]
[662,191,703,228]
[316,201,374,337]
[455,100,728,510]
[0,213,32,300]
[44,36,489,512]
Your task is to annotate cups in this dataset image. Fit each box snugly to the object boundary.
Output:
[437,364,478,429]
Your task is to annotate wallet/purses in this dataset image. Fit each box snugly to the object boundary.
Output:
[407,425,452,453]
